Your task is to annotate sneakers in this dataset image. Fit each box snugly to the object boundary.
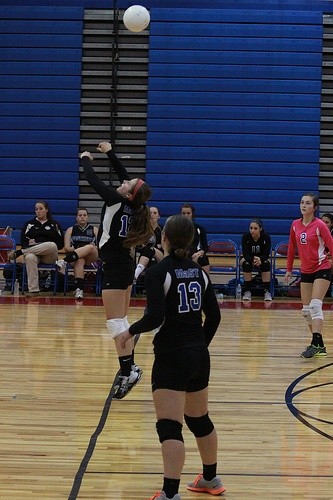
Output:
[263,289,273,301]
[75,288,84,299]
[242,291,252,301]
[149,490,181,500]
[113,364,143,389]
[186,472,226,495]
[300,344,320,358]
[315,345,328,356]
[54,259,68,271]
[113,370,141,399]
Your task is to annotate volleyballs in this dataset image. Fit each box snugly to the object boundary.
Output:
[123,5,151,33]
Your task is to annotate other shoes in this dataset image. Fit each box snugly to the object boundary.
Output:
[26,291,40,297]
[7,250,19,260]
[133,276,137,285]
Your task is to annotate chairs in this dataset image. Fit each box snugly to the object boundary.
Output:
[64,259,103,295]
[0,235,22,294]
[238,243,273,297]
[21,261,61,295]
[134,243,144,297]
[205,238,238,300]
[273,240,300,300]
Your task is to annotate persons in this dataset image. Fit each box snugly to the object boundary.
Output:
[7,200,64,296]
[242,218,272,301]
[81,142,154,399]
[121,215,226,500]
[133,206,164,285]
[322,214,333,237]
[284,194,333,360]
[181,203,210,276]
[64,207,99,300]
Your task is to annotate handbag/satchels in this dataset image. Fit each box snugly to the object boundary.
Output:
[3,263,29,291]
